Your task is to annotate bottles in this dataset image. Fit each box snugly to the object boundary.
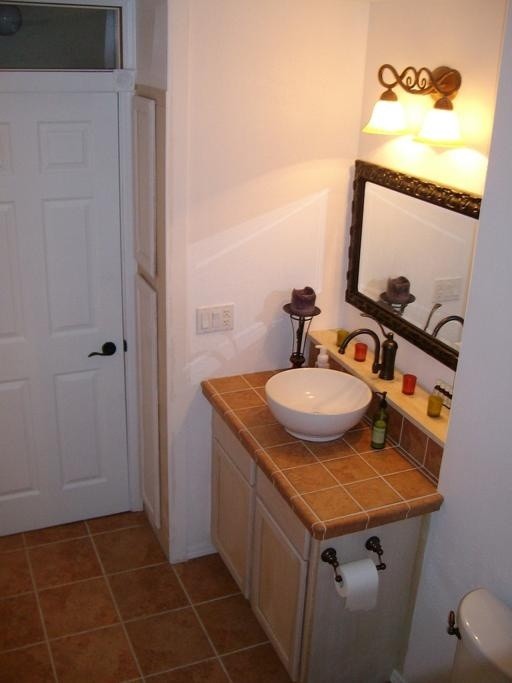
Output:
[432,381,453,410]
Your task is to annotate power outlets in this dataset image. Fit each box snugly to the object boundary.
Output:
[221,303,234,330]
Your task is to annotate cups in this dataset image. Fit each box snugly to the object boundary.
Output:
[337,330,349,347]
[354,343,368,361]
[427,396,442,418]
[403,374,416,395]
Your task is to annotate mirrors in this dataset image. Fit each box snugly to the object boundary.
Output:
[344,158,483,370]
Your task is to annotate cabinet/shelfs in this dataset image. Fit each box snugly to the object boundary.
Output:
[209,407,424,682]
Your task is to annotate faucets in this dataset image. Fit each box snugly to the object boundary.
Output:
[339,313,399,380]
[423,303,465,337]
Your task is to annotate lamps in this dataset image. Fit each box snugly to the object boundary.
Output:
[360,63,463,147]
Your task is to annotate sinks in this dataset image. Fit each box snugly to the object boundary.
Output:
[265,367,373,441]
